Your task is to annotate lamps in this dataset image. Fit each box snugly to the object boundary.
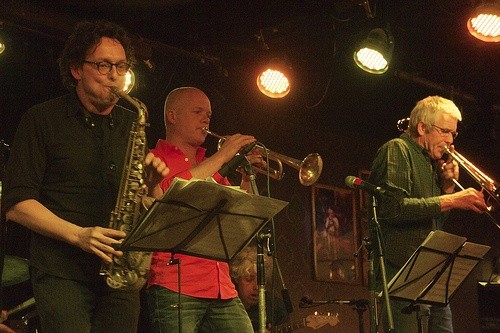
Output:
[465,0,500,45]
[353,24,394,74]
[122,62,149,95]
[257,57,292,98]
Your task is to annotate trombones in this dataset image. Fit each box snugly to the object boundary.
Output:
[396,117,500,229]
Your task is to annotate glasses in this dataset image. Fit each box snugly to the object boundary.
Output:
[431,122,459,139]
[79,55,130,76]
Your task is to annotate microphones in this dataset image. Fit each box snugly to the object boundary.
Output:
[207,141,256,184]
[299,296,309,308]
[345,176,393,197]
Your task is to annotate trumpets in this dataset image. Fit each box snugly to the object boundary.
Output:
[199,126,322,187]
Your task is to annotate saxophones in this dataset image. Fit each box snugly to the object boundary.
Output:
[98,86,149,291]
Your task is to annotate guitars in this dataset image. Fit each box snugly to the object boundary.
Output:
[266,311,340,333]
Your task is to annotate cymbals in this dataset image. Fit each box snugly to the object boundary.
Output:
[0,254,31,290]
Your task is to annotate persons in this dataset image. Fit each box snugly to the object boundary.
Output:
[140,86,267,333]
[229,246,290,333]
[326,208,341,265]
[1,19,170,333]
[363,96,488,333]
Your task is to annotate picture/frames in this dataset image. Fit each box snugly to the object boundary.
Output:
[309,182,366,288]
